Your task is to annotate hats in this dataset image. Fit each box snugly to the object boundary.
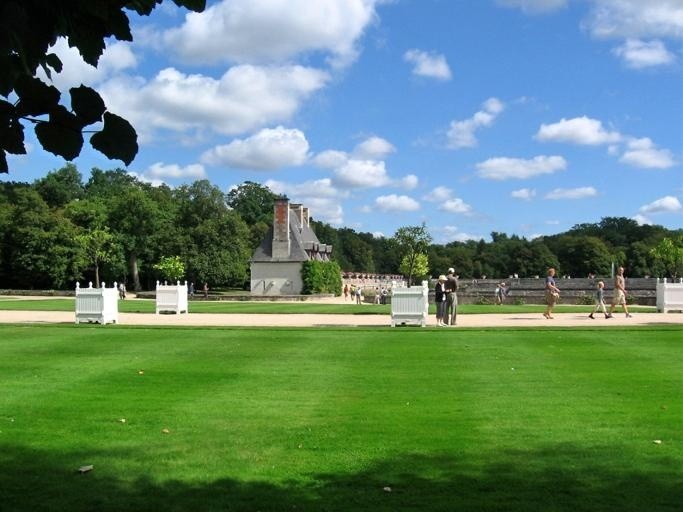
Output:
[448,268,454,273]
[437,275,448,281]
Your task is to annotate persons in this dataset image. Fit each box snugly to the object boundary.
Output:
[498,282,507,304]
[587,281,612,319]
[189,282,194,301]
[118,283,127,299]
[198,282,209,301]
[494,283,500,306]
[608,266,632,317]
[435,275,453,326]
[445,268,458,325]
[343,283,387,305]
[542,268,560,319]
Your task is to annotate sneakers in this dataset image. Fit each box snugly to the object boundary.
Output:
[626,314,631,318]
[544,312,550,318]
[437,322,446,326]
[589,315,594,319]
[606,314,612,319]
